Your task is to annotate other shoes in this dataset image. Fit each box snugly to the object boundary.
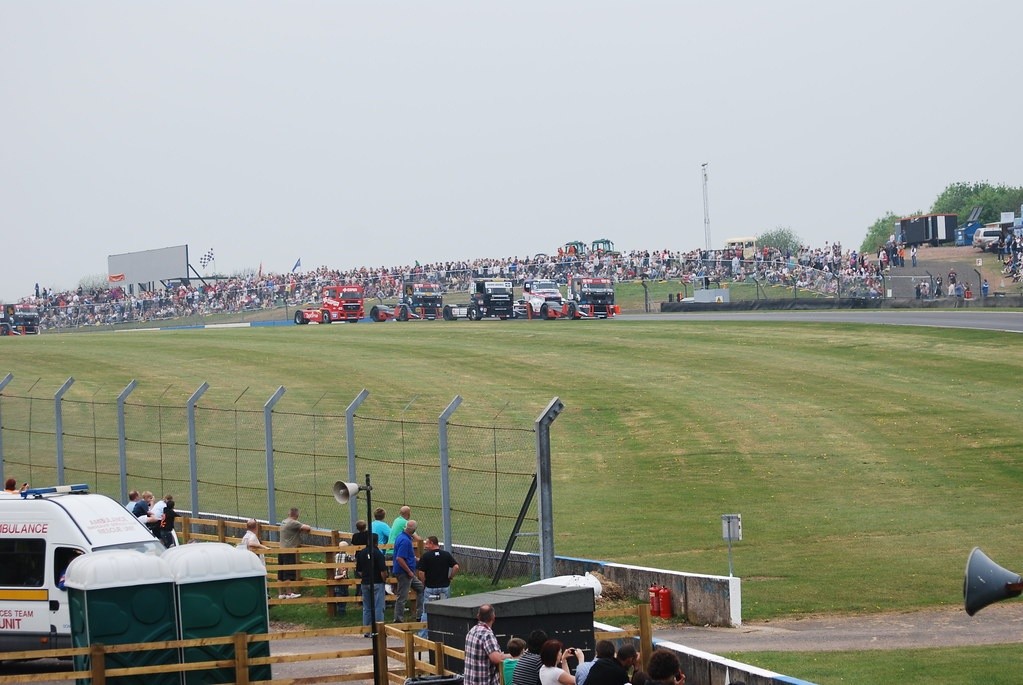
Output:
[364,633,373,638]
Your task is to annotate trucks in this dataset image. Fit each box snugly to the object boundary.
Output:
[567,273,620,319]
[0,303,41,336]
[441,277,515,321]
[512,278,569,320]
[369,280,444,322]
[293,285,365,323]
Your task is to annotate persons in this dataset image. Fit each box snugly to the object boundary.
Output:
[914,230,1023,299]
[187,538,198,544]
[241,517,276,627]
[662,241,917,297]
[278,506,310,599]
[322,260,478,298]
[463,603,746,685]
[3,478,29,494]
[203,272,346,313]
[476,245,661,287]
[126,490,179,548]
[333,541,356,618]
[352,505,459,638]
[20,283,210,324]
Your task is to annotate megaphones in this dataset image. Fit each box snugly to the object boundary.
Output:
[334,480,361,504]
[963,546,1023,616]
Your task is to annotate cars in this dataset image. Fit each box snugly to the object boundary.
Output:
[985,239,999,254]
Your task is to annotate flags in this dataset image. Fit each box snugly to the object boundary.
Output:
[199,248,213,269]
[258,263,263,277]
[292,258,300,274]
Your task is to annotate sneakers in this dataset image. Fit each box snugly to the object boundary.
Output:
[384,584,394,595]
[279,594,287,599]
[286,593,301,599]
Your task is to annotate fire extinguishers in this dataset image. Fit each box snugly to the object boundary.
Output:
[649,583,661,617]
[659,585,672,618]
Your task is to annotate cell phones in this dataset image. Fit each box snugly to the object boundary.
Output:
[571,649,576,654]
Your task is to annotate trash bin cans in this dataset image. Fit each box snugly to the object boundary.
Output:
[404,674,464,685]
[669,294,674,302]
[160,542,272,685]
[64,549,182,685]
[966,291,972,299]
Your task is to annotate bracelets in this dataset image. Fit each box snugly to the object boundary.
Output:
[450,577,453,579]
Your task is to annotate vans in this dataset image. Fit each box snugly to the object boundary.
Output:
[971,227,1003,251]
[0,483,171,666]
